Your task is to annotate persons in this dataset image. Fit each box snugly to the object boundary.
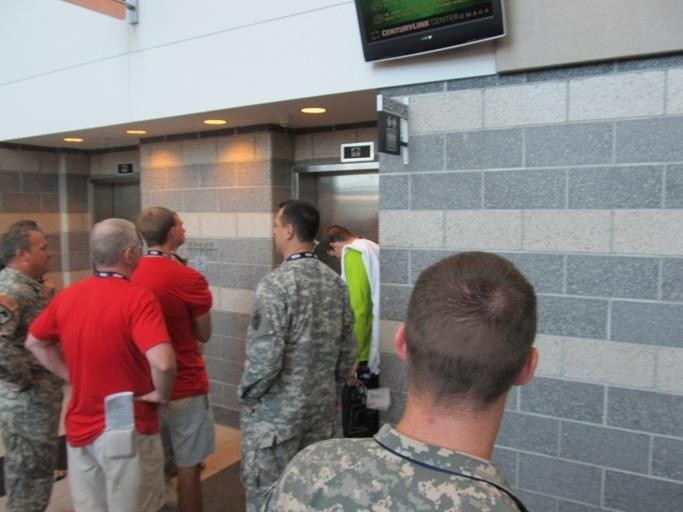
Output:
[252,248,540,511]
[136,203,215,510]
[234,198,359,512]
[25,220,184,510]
[0,215,60,512]
[320,224,380,380]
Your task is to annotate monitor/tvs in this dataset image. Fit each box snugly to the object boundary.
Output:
[355,0,509,63]
